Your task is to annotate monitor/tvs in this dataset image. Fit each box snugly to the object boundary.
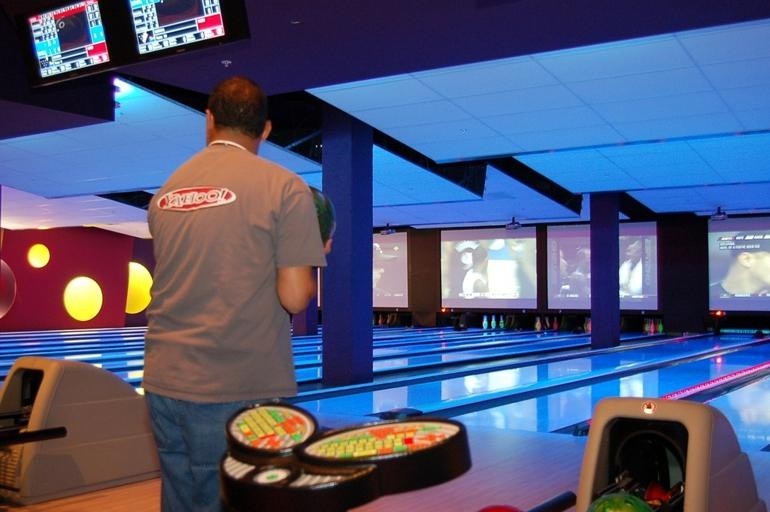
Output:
[115,0,250,67]
[20,0,125,88]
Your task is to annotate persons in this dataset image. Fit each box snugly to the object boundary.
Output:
[619,238,644,300]
[709,232,770,298]
[371,243,400,297]
[141,74,336,511]
[462,246,492,300]
[568,249,591,299]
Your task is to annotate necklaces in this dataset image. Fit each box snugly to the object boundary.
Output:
[207,139,247,150]
